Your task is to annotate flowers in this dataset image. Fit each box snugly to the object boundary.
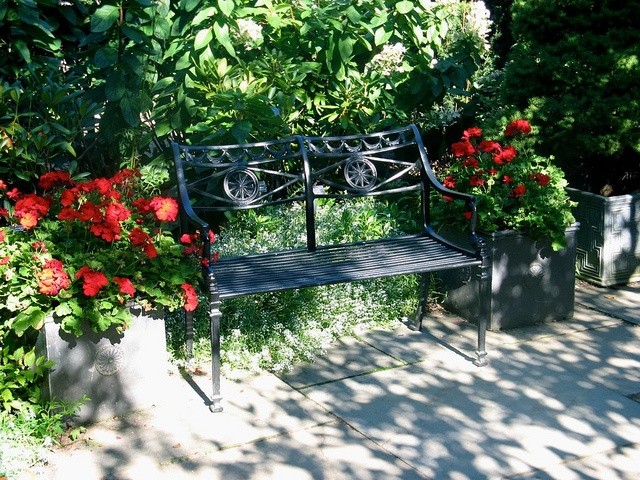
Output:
[0,170,220,339]
[433,108,579,251]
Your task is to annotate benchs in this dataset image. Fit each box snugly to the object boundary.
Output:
[169,121,494,412]
